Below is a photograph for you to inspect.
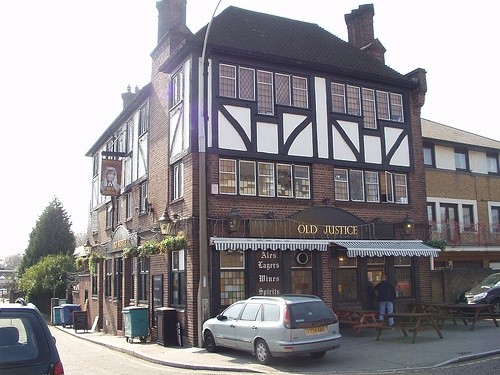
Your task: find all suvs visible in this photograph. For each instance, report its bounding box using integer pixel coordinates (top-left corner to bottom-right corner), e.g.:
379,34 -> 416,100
0,297 -> 64,375
202,292 -> 343,364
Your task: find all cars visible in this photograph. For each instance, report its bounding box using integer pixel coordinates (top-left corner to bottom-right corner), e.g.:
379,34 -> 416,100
464,272 -> 500,321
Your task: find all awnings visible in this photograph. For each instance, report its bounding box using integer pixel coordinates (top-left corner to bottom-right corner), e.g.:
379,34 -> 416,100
213,240 -> 328,252
334,242 -> 441,257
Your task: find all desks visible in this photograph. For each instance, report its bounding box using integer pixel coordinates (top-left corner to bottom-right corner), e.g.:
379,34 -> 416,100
333,307 -> 380,336
375,312 -> 444,345
437,302 -> 500,332
408,302 -> 458,325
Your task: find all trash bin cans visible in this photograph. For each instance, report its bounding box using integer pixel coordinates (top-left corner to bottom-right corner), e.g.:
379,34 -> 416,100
60,304 -> 80,325
121,306 -> 149,336
155,307 -> 177,346
53,307 -> 61,325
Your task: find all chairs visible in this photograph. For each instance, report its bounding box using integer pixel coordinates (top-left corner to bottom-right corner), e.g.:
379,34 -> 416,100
0,326 -> 32,362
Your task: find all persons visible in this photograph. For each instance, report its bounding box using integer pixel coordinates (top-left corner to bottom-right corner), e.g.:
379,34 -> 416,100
372,275 -> 395,326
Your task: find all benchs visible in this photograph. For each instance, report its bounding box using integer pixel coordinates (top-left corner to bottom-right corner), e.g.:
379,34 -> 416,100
338,319 -> 360,330
462,311 -> 499,316
366,319 -> 385,329
376,323 -> 419,333
435,315 -> 478,320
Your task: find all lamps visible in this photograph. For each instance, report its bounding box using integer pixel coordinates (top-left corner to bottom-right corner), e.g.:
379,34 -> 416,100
224,203 -> 244,235
401,212 -> 416,240
157,205 -> 174,235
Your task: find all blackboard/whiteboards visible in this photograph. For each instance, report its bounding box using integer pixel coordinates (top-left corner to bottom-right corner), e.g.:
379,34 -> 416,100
72,310 -> 87,330
